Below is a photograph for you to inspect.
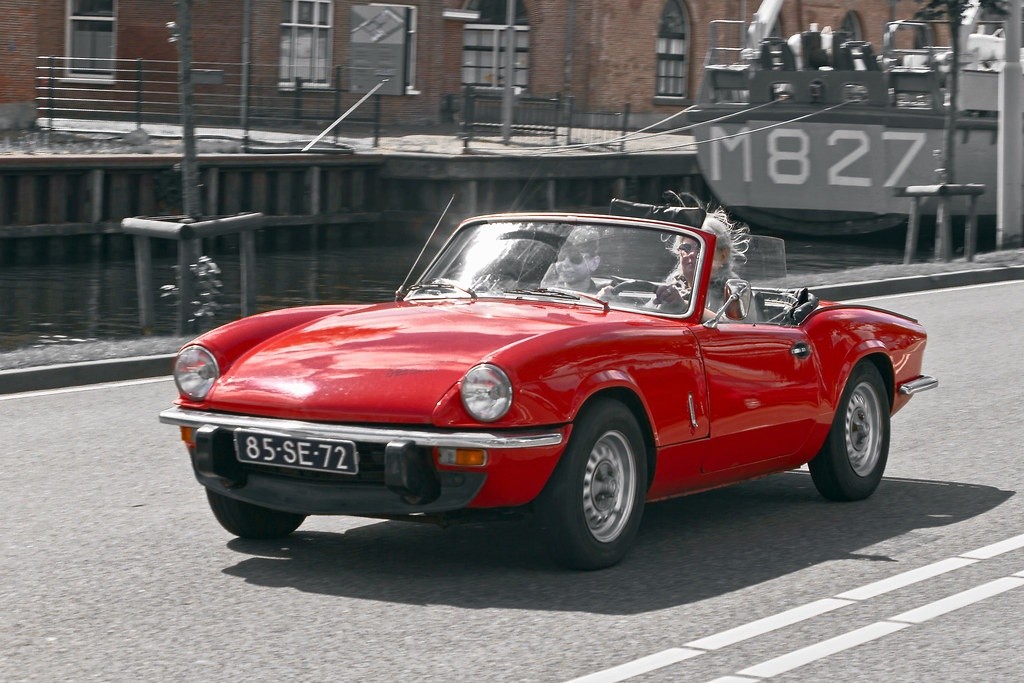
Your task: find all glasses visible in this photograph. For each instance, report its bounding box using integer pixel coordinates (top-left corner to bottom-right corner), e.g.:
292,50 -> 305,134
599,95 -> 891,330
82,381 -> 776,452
553,251 -> 592,264
677,244 -> 701,254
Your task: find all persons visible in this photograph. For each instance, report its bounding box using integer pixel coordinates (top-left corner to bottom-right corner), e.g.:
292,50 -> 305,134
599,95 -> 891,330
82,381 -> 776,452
598,212 -> 756,324
550,225 -> 604,294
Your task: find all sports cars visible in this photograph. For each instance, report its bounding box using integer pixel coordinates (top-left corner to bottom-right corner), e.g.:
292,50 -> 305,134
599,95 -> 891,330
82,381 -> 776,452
156,198 -> 938,572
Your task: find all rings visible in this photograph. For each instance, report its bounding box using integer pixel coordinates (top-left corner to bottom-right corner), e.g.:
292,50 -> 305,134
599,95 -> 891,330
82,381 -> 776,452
666,289 -> 672,293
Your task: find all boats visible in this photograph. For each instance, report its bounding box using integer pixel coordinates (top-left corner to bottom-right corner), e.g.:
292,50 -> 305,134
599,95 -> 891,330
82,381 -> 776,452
684,0 -> 1024,239
